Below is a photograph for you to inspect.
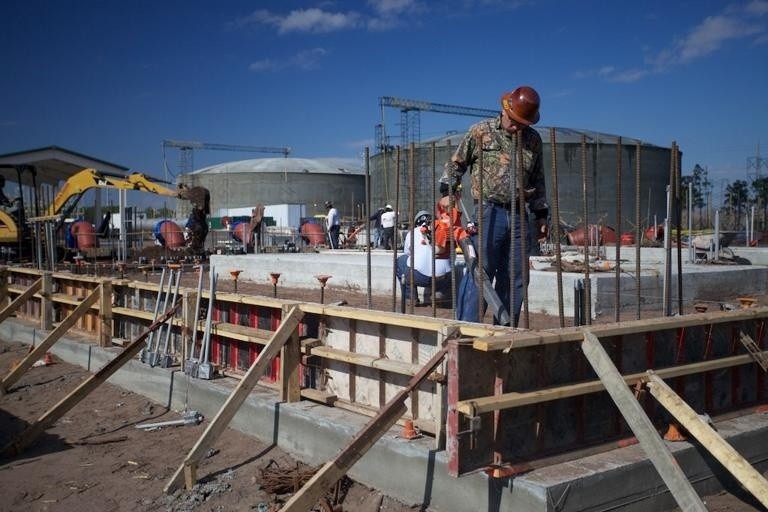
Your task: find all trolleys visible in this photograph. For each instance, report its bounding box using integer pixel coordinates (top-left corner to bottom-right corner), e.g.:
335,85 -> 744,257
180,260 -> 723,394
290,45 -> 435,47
675,231 -> 737,262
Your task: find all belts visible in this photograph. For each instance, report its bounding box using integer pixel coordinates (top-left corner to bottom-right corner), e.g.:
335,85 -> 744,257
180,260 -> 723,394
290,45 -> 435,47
472,198 -> 522,210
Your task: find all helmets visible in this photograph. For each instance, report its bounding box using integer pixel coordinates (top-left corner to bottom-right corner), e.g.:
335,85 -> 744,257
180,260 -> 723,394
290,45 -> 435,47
385,204 -> 393,210
500,86 -> 540,127
324,200 -> 333,209
414,210 -> 432,226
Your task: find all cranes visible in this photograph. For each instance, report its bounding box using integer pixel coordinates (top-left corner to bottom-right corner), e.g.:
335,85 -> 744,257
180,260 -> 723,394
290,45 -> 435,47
155,138 -> 296,222
376,95 -> 505,232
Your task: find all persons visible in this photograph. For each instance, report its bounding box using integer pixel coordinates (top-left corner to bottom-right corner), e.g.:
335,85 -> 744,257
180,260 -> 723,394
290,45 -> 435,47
437,85 -> 551,328
324,200 -> 342,249
368,207 -> 386,249
380,204 -> 401,250
393,210 -> 465,308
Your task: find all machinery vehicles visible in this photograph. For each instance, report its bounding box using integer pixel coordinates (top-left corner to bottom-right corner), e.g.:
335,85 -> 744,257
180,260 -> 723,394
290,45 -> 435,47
0,161 -> 211,262
